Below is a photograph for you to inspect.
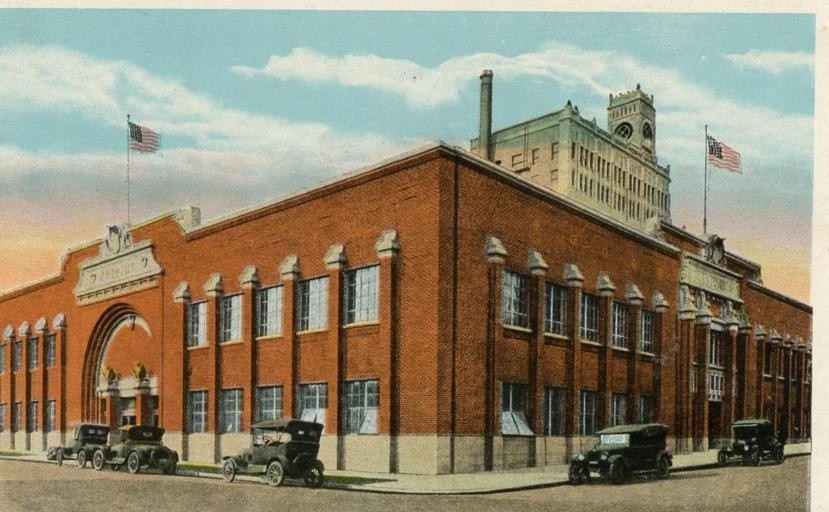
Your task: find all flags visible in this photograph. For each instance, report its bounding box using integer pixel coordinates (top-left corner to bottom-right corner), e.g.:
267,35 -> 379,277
126,117 -> 164,159
703,122 -> 743,176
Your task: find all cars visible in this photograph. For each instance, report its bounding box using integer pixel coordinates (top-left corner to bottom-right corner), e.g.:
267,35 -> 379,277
567,418 -> 672,485
44,422 -> 110,468
90,421 -> 179,476
716,419 -> 787,469
220,417 -> 325,490
